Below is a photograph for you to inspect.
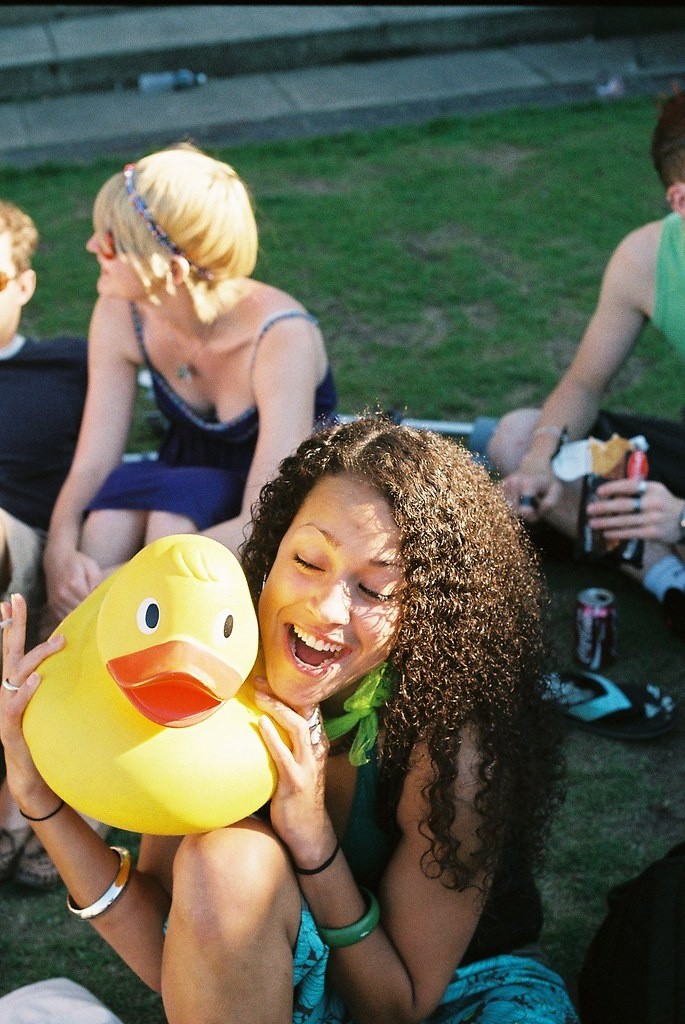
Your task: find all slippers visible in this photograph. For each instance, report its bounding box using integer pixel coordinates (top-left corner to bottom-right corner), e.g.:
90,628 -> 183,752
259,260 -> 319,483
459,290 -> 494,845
558,669 -> 678,740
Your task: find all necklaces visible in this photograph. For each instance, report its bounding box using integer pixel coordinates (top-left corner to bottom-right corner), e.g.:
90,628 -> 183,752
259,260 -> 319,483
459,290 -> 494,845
174,357 -> 197,382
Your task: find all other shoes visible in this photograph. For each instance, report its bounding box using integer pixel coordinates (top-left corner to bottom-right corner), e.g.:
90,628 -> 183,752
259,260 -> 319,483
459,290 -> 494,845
0,817 -> 112,888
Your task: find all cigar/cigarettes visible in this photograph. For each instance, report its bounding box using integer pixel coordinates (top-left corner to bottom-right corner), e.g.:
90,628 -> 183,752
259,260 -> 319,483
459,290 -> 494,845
0,617 -> 12,628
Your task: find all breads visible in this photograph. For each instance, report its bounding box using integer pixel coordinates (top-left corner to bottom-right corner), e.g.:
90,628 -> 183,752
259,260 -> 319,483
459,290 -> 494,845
586,434 -> 637,481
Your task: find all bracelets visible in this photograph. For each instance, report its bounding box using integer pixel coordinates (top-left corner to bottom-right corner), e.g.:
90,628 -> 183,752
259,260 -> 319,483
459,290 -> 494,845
318,887 -> 380,949
530,427 -> 568,443
20,800 -> 65,822
294,840 -> 340,875
66,845 -> 134,922
678,507 -> 685,545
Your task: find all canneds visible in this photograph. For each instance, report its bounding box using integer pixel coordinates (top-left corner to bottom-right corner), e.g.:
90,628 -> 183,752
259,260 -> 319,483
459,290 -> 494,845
574,587 -> 617,671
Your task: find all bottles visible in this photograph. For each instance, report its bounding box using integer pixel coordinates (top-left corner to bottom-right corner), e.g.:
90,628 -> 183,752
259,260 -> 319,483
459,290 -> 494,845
139,68 -> 208,94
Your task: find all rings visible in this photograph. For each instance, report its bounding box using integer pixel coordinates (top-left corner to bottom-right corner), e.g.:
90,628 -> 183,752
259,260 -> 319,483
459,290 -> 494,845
638,481 -> 648,495
307,708 -> 323,746
634,498 -> 641,512
519,495 -> 535,506
3,679 -> 19,692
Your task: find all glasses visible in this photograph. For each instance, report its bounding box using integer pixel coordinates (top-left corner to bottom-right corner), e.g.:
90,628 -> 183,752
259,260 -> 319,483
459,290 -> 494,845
0,271 -> 17,292
95,229 -> 135,260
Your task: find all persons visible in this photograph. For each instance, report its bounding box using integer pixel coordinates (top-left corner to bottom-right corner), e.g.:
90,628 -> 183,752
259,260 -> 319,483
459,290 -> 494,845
0,419 -> 568,1024
42,148 -> 338,623
0,201 -> 90,604
488,89 -> 685,612
